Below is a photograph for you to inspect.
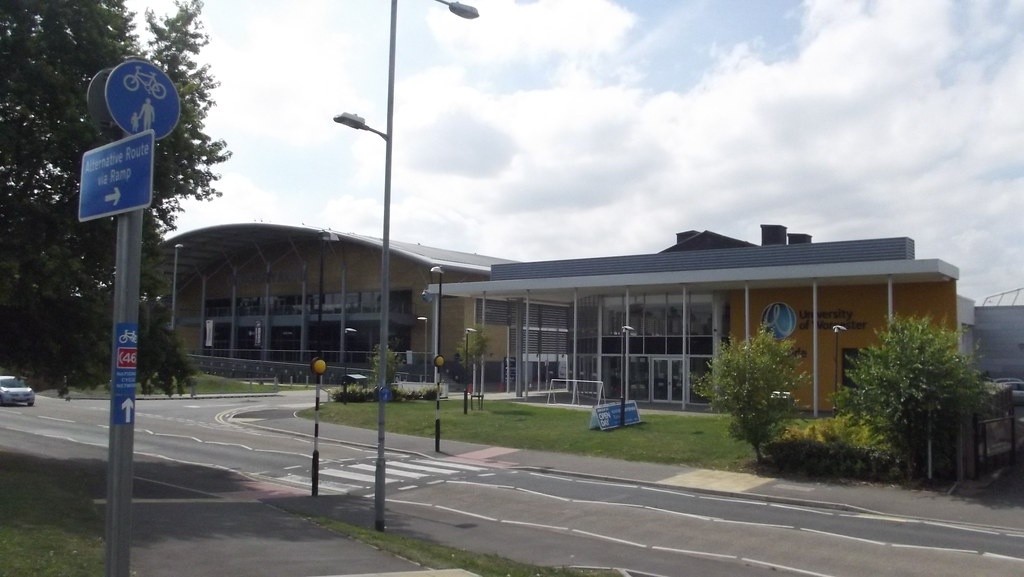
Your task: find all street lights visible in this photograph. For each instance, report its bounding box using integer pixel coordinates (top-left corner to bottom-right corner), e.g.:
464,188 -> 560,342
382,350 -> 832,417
430,267 -> 444,452
170,244 -> 184,330
331,0 -> 479,534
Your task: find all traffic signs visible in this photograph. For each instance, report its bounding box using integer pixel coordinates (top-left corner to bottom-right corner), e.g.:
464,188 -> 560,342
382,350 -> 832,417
78,129 -> 154,223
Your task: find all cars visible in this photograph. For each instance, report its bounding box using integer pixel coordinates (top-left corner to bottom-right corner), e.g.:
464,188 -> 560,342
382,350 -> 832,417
0,376 -> 36,406
